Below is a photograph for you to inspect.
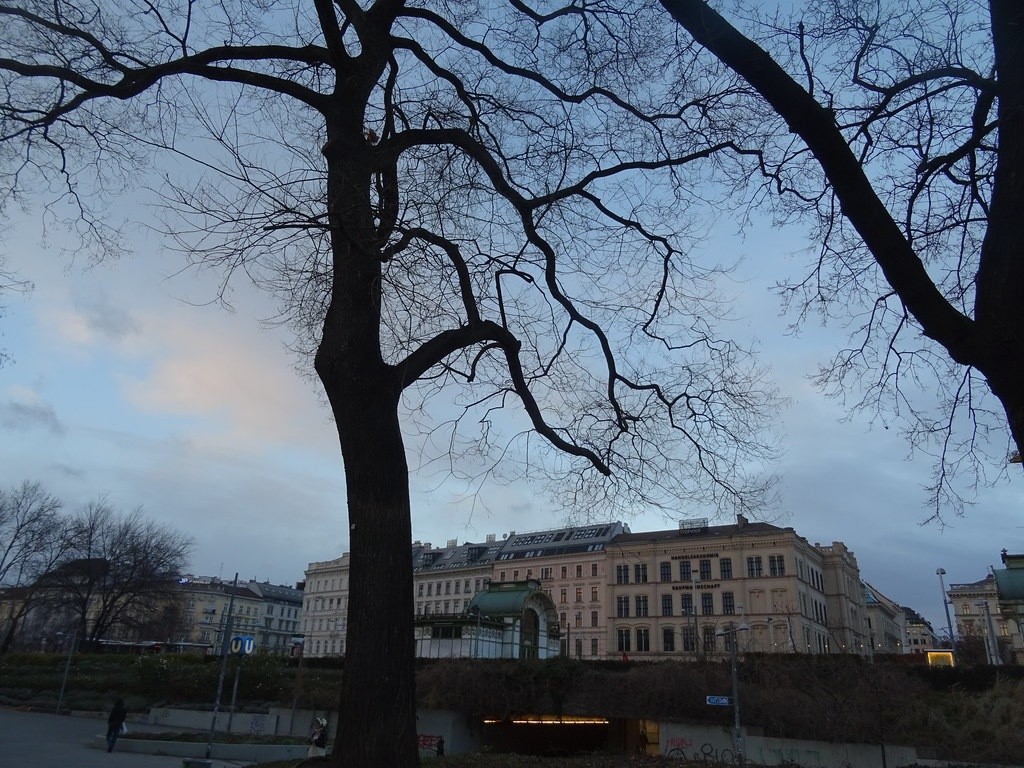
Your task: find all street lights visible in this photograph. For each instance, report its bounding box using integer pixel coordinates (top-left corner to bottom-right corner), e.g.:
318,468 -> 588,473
680,609 -> 694,662
936,567 -> 962,664
974,602 -> 999,665
714,624 -> 750,768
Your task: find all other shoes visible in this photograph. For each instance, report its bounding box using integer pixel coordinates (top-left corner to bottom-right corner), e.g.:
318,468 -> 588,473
107,750 -> 113,752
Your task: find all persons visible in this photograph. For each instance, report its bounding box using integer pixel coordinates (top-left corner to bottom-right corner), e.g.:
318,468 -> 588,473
633,745 -> 640,761
307,717 -> 329,759
106,698 -> 127,753
640,730 -> 650,753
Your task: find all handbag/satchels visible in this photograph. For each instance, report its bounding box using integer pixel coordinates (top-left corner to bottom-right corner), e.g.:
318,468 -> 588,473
119,722 -> 127,735
